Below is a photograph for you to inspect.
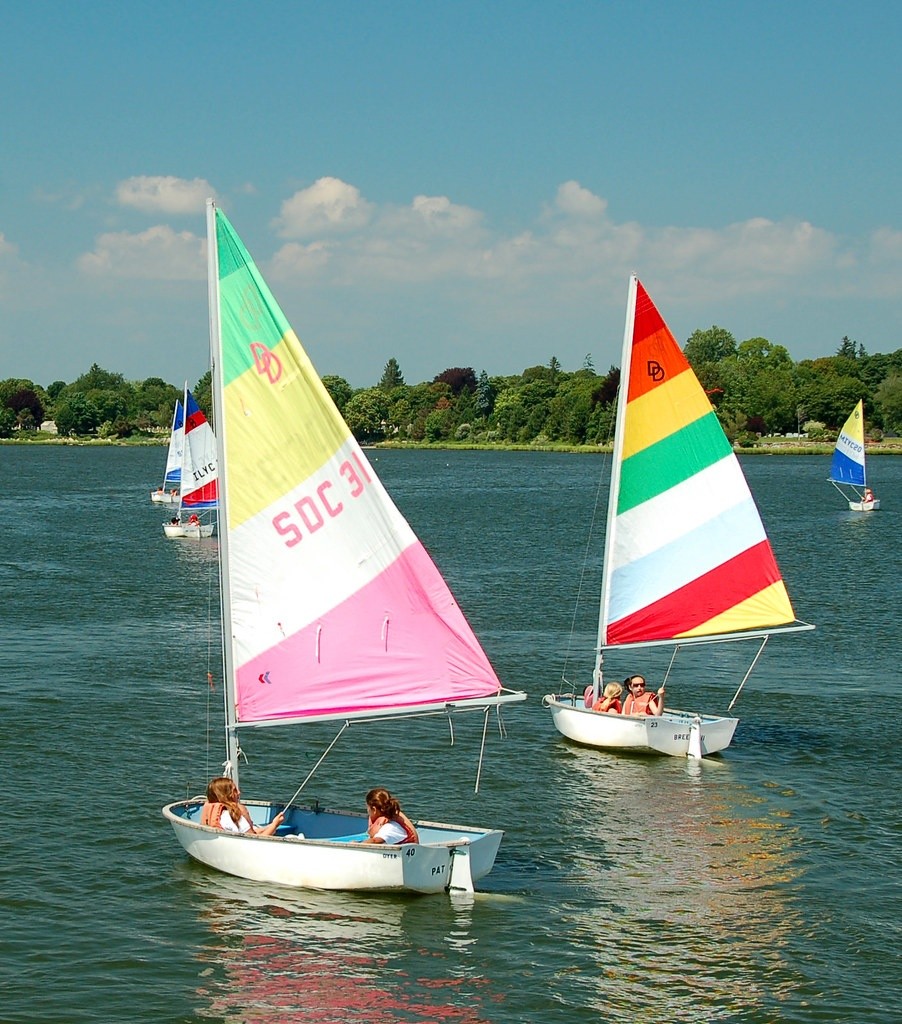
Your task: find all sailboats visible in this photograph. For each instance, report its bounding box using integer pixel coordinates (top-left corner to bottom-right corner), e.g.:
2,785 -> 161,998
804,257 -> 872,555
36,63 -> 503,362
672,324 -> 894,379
162,380 -> 216,537
826,398 -> 881,511
160,199 -> 527,891
539,272 -> 815,765
151,398 -> 184,504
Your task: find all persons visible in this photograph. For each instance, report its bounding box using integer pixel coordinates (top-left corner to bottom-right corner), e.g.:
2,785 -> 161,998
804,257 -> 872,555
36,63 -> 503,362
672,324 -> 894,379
350,787 -> 420,845
170,489 -> 177,496
592,682 -> 622,714
864,489 -> 874,503
157,487 -> 163,495
188,514 -> 200,526
171,517 -> 181,525
621,675 -> 665,716
201,777 -> 284,836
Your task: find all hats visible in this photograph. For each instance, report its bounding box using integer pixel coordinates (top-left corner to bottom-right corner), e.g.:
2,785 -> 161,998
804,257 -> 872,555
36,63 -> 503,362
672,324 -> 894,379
866,488 -> 871,493
171,517 -> 180,523
189,514 -> 198,521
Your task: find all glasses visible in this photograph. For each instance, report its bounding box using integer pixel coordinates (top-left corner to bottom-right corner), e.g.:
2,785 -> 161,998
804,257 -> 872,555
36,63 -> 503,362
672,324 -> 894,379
631,682 -> 645,687
